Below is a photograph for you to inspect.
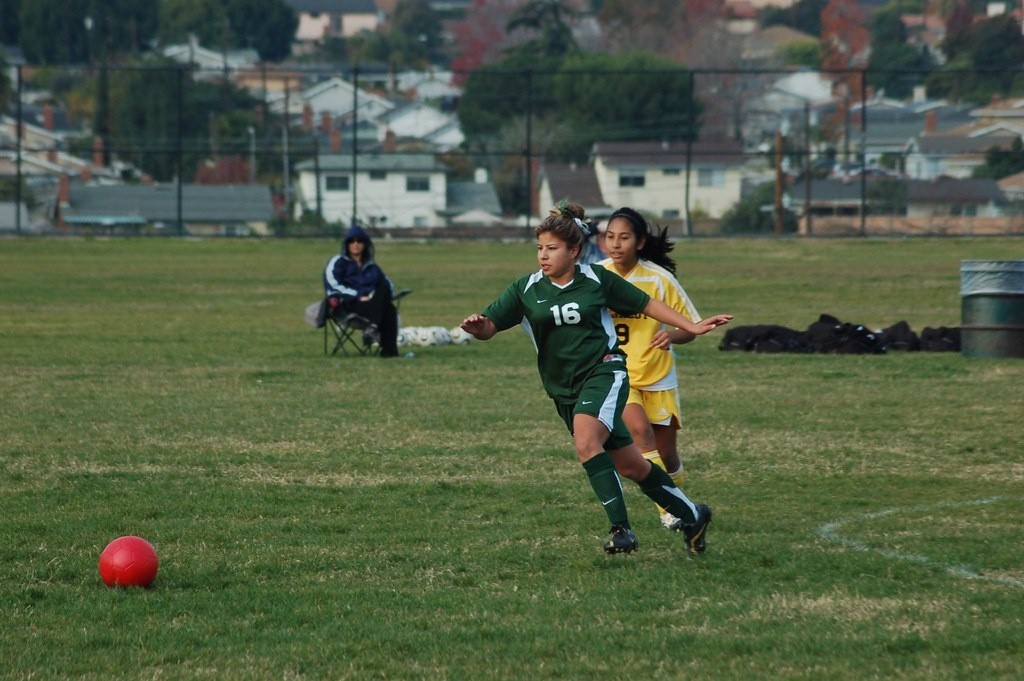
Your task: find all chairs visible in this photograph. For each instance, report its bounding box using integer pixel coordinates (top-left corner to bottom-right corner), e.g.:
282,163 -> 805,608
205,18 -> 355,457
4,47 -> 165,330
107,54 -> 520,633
323,269 -> 400,356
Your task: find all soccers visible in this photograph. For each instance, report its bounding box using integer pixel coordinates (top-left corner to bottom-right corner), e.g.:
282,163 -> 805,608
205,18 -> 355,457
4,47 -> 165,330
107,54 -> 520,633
450,326 -> 474,347
397,326 -> 449,346
100,536 -> 159,586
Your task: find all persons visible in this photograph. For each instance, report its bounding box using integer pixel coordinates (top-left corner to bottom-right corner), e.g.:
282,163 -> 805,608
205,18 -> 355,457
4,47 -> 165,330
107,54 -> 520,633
322,224 -> 401,358
591,206 -> 701,531
460,203 -> 734,559
578,218 -> 611,267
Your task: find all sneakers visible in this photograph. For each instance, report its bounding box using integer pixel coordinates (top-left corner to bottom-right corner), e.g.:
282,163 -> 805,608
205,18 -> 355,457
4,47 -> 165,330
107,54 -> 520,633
603,524 -> 638,554
680,503 -> 711,557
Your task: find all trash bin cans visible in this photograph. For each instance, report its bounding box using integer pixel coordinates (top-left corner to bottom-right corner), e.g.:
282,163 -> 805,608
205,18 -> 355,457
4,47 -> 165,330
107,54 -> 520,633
960,259 -> 1024,360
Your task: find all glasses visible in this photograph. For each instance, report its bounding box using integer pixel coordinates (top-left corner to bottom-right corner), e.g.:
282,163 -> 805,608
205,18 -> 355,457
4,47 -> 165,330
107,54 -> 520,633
348,239 -> 362,244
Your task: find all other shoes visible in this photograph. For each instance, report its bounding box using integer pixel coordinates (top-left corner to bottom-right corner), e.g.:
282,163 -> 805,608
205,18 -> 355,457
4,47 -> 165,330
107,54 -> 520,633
362,330 -> 373,346
661,513 -> 682,528
